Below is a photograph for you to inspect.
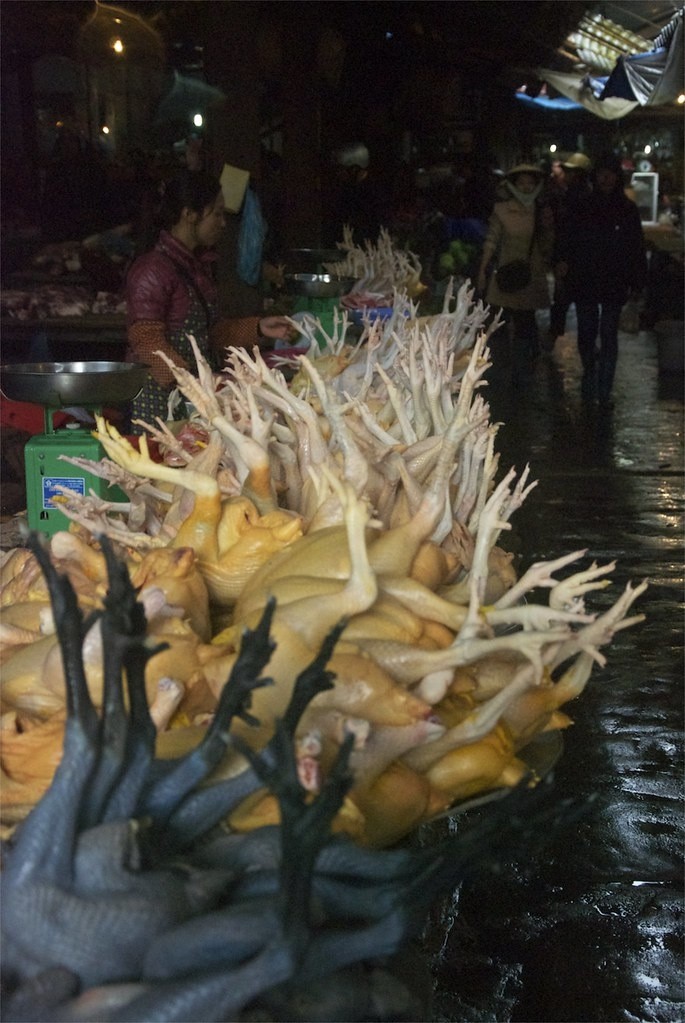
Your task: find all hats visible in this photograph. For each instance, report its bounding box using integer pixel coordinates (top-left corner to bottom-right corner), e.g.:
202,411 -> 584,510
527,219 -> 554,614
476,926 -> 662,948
507,164 -> 542,174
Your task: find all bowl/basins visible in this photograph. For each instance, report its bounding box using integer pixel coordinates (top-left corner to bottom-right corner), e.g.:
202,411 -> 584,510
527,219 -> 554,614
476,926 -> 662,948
354,307 -> 407,326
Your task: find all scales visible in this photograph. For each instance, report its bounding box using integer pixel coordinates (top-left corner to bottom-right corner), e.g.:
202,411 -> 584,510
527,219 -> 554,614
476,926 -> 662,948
285,246 -> 350,314
0,358 -> 151,541
283,272 -> 364,355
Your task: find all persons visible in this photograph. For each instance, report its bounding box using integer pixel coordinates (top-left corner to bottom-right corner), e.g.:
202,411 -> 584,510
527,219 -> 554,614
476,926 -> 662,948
35,132 -> 615,271
559,154 -> 650,412
475,160 -> 553,384
122,172 -> 292,454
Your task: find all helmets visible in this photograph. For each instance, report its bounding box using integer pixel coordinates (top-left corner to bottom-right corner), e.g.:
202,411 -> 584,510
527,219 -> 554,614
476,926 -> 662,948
332,139 -> 369,172
563,152 -> 590,170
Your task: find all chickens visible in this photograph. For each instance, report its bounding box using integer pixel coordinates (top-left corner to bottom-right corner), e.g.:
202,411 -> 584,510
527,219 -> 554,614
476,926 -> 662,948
0,222 -> 650,1023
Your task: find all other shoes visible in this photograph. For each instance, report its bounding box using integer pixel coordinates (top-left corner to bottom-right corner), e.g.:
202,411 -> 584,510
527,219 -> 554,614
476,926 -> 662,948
579,377 -> 616,412
542,330 -> 559,352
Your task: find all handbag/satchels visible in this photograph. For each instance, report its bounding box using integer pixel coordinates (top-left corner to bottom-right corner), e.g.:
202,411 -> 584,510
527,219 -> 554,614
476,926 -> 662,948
496,260 -> 532,291
